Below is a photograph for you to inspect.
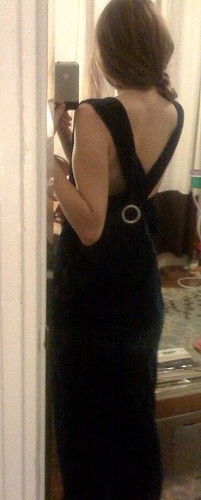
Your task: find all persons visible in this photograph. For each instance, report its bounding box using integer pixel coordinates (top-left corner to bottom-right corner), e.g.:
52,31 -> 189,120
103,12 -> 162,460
47,1 -> 185,499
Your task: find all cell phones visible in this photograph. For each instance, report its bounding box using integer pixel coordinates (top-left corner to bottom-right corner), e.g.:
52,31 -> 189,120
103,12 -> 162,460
54,61 -> 79,110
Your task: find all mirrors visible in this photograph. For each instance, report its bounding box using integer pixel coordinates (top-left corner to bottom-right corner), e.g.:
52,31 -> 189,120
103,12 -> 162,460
43,1 -> 201,500
